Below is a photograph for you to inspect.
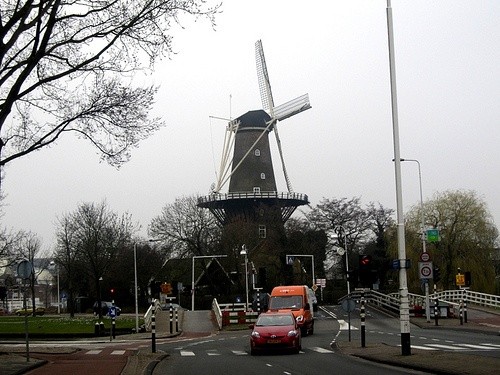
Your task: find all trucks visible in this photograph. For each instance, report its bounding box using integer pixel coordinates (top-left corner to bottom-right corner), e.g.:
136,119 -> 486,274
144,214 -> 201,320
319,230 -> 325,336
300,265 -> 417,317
266,286 -> 316,337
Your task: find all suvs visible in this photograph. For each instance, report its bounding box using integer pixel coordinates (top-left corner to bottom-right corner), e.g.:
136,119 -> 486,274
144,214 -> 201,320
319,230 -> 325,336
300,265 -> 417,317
93,301 -> 121,316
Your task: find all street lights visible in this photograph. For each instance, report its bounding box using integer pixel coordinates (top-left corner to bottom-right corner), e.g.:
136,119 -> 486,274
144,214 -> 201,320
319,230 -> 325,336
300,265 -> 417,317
239,244 -> 250,311
392,158 -> 433,323
133,239 -> 160,332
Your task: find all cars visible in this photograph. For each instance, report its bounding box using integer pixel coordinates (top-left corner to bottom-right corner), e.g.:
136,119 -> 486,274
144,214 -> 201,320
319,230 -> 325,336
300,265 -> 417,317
248,312 -> 302,354
309,289 -> 317,305
15,304 -> 46,316
0,308 -> 12,315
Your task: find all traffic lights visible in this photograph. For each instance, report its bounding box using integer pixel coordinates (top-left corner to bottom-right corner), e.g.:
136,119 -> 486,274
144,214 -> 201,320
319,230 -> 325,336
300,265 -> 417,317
433,266 -> 441,284
349,251 -> 379,279
